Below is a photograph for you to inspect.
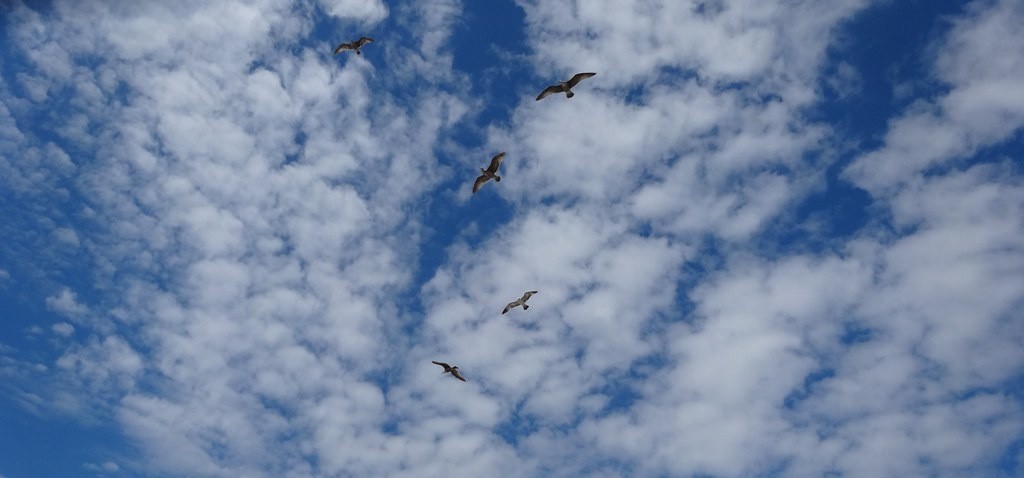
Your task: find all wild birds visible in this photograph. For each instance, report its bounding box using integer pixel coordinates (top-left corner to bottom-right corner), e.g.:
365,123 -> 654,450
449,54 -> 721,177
536,71 -> 596,103
334,38 -> 375,57
430,291 -> 544,383
473,151 -> 508,193
